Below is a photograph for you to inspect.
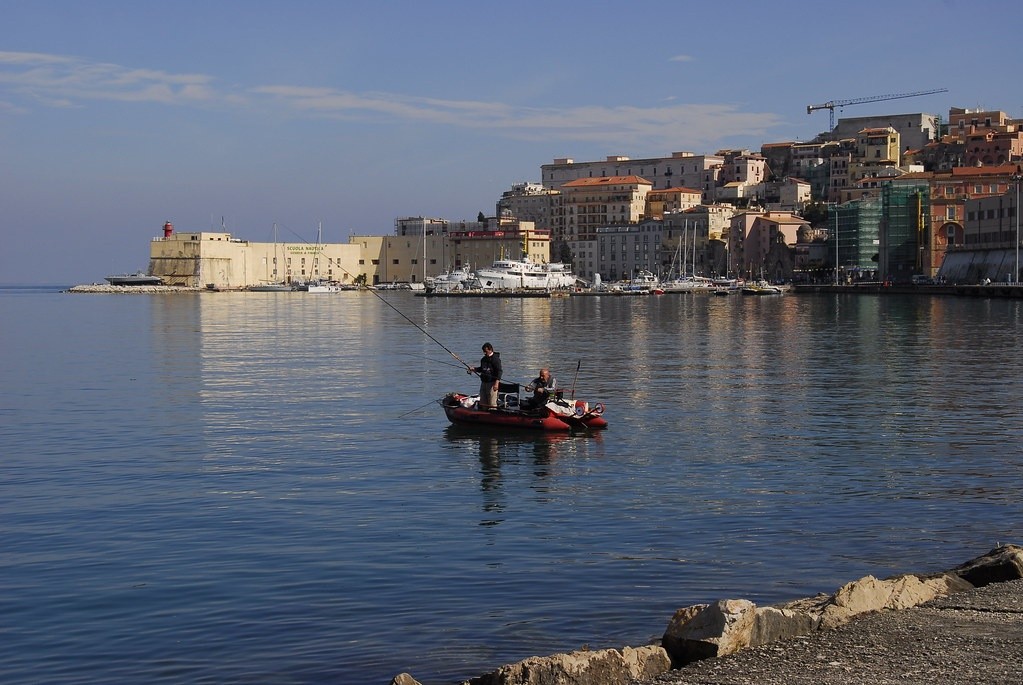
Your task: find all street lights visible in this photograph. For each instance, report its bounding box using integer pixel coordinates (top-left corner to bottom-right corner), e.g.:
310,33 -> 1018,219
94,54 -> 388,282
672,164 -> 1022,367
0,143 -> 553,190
831,202 -> 840,285
1009,174 -> 1023,284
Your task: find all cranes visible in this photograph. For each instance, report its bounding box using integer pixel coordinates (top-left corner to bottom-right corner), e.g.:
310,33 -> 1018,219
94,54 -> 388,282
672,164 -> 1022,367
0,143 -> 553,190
807,87 -> 950,134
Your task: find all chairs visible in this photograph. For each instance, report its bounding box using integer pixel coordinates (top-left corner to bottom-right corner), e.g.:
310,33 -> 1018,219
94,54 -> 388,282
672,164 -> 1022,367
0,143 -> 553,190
498,382 -> 520,408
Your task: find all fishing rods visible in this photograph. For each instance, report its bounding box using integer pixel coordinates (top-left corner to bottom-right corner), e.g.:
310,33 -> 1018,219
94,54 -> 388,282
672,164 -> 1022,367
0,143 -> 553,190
383,349 -> 535,392
277,223 -> 481,380
397,393 -> 454,419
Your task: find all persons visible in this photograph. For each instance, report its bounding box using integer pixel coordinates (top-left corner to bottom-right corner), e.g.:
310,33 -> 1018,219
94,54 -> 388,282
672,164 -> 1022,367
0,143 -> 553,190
468,343 -> 502,411
520,368 -> 557,418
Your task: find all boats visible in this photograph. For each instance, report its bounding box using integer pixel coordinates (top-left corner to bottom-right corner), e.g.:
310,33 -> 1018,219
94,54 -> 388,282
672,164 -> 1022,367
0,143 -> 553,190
248,217 -> 794,300
104,268 -> 164,285
435,358 -> 609,434
